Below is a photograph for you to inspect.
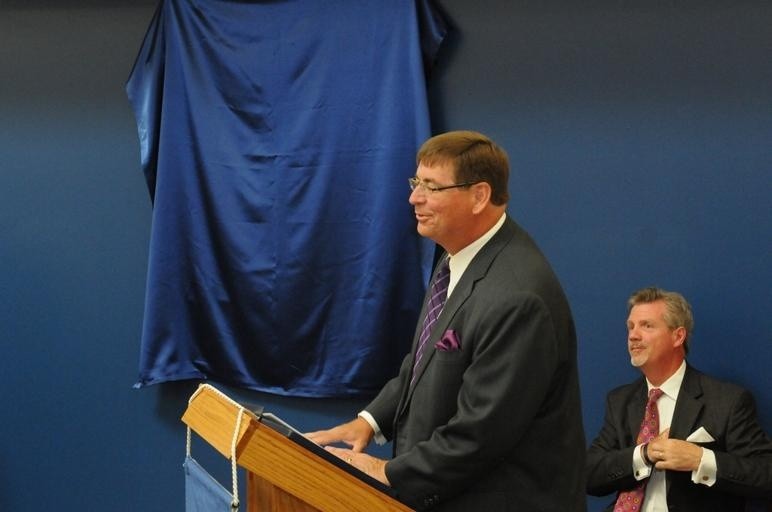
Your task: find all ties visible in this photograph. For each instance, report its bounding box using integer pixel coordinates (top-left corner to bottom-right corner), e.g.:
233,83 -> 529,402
613,388 -> 663,512
409,257 -> 451,391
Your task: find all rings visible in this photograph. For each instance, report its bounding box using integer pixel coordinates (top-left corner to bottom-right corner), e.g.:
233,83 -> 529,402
658,451 -> 664,460
347,457 -> 352,465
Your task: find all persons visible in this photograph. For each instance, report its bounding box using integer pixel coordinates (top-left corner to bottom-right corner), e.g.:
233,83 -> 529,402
303,131 -> 588,511
584,288 -> 771,511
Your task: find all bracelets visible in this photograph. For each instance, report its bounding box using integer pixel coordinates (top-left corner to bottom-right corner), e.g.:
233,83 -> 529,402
643,441 -> 655,466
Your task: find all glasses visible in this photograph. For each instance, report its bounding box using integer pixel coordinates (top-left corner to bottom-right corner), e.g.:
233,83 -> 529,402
408,177 -> 474,197
